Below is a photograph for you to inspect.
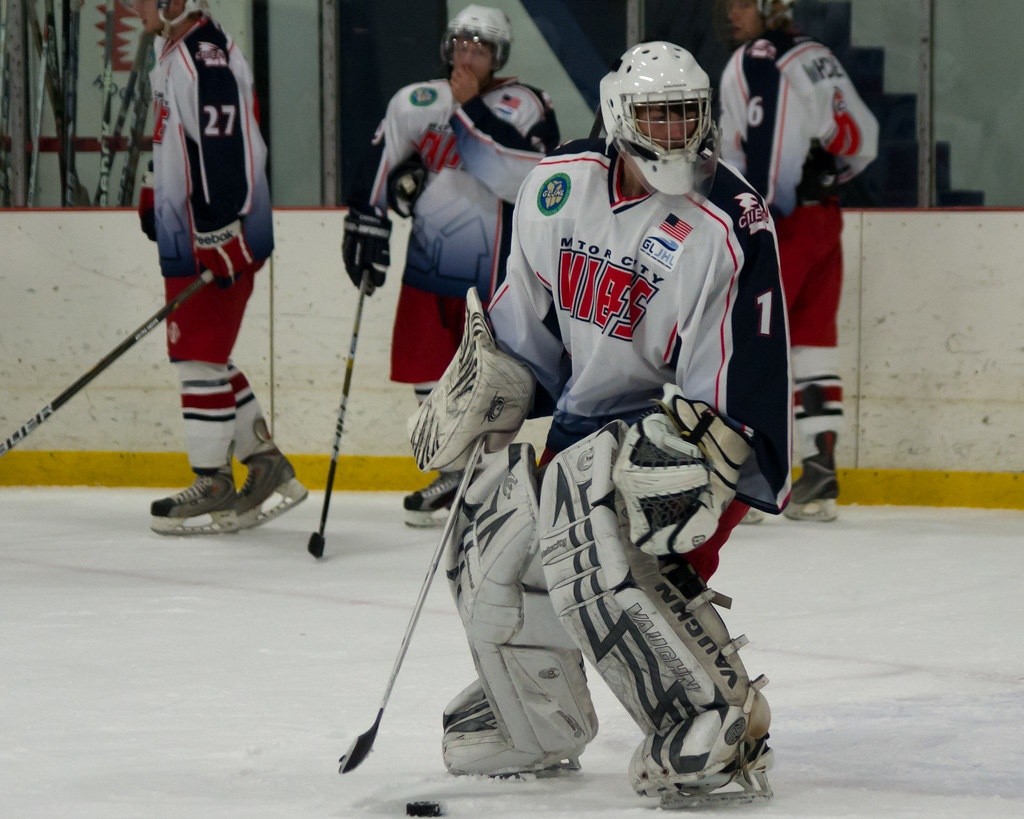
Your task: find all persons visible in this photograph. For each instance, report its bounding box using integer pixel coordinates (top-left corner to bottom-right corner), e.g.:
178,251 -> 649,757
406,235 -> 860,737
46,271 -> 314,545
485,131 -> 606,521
131,0 -> 311,535
719,0 -> 881,522
342,3 -> 560,530
408,42 -> 792,812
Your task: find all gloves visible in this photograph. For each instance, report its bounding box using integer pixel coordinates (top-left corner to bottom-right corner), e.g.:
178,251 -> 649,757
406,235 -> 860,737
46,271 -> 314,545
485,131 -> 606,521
342,208 -> 392,296
386,151 -> 426,218
188,219 -> 256,288
794,137 -> 850,207
138,159 -> 157,241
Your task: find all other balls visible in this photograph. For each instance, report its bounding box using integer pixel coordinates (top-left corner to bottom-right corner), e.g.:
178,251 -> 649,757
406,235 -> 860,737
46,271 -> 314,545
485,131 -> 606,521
406,801 -> 440,817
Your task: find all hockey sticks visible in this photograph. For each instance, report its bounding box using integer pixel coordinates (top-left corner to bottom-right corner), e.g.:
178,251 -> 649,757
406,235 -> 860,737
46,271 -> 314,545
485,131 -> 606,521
336,430 -> 489,775
0,268 -> 216,457
307,266 -> 371,560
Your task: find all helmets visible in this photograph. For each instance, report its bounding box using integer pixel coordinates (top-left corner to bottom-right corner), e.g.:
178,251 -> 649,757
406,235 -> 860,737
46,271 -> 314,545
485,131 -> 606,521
600,40 -> 713,196
755,0 -> 796,21
447,4 -> 513,72
153,0 -> 203,26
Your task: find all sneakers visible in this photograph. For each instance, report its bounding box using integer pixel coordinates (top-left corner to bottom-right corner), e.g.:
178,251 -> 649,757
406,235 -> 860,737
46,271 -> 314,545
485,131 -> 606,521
782,430 -> 840,521
739,507 -> 764,524
644,732 -> 774,809
235,416 -> 308,529
488,746 -> 582,780
149,440 -> 242,536
403,472 -> 473,526
